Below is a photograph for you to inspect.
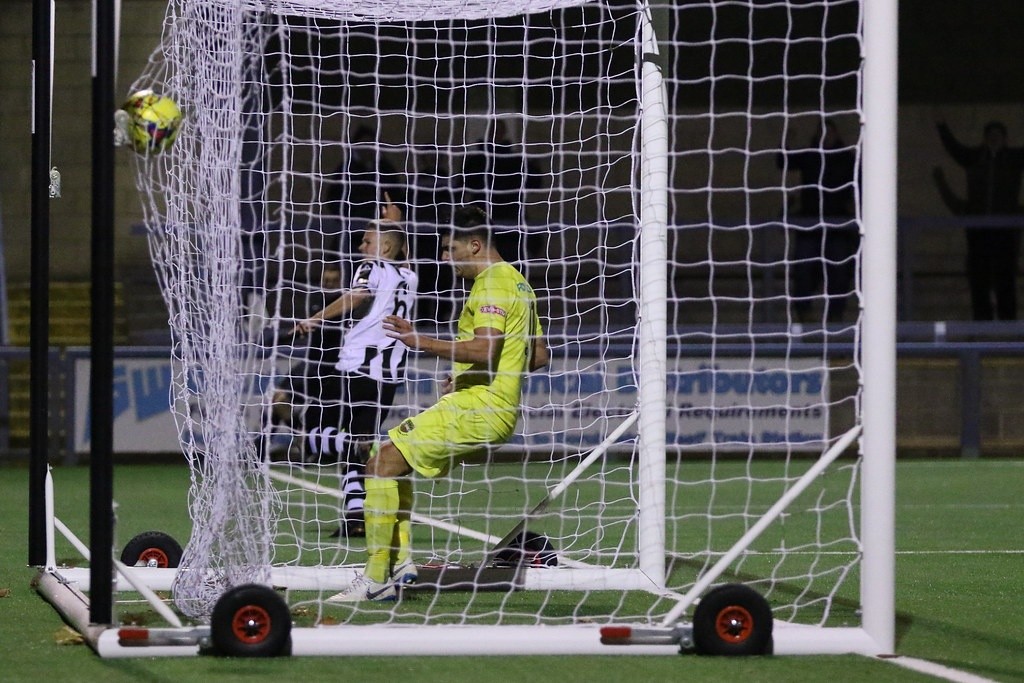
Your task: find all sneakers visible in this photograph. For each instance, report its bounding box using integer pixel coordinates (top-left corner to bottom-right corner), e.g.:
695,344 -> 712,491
330,512 -> 366,536
326,569 -> 397,602
352,557 -> 417,591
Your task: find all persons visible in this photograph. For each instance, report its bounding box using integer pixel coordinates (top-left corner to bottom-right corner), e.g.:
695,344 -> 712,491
328,206 -> 546,602
323,120 -> 544,260
308,267 -> 353,360
777,118 -> 855,319
929,103 -> 1023,322
242,191 -> 420,538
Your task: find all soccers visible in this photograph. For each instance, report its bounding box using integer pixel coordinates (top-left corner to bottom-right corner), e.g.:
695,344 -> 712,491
120,89 -> 182,157
